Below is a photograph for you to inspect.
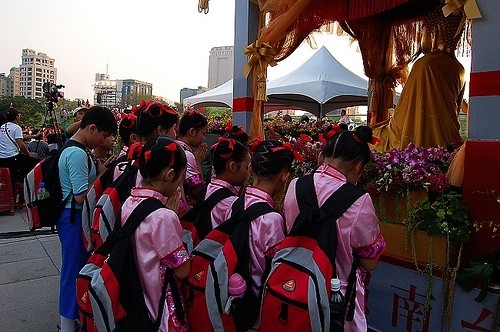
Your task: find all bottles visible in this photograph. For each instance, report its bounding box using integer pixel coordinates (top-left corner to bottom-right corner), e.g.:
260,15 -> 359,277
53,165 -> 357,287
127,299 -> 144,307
229,273 -> 247,299
329,278 -> 346,332
37,182 -> 46,200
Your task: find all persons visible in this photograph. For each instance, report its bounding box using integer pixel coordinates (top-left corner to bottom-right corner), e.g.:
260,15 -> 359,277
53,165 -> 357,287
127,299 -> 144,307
27,129 -> 58,160
0,107 -> 38,169
77,99 -> 89,107
284,124 -> 387,332
368,0 -> 467,153
113,99 -> 208,218
121,137 -> 190,332
203,136 -> 251,229
339,110 -> 350,125
55,106 -> 118,332
224,136 -> 306,297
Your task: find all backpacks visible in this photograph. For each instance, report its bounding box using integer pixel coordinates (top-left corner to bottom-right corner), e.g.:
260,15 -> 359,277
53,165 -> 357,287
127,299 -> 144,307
24,140 -> 92,232
180,183 -> 284,332
81,153 -> 141,254
76,198 -> 184,332
258,172 -> 367,332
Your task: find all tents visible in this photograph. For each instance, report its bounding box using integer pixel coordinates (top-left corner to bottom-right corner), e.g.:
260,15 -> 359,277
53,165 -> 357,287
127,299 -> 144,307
183,46 -> 400,119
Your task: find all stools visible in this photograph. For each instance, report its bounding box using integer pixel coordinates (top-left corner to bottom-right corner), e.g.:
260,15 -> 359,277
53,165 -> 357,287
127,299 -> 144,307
0,168 -> 15,216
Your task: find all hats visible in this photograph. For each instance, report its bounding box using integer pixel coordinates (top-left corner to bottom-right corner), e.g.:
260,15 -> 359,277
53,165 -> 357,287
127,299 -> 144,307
72,107 -> 89,116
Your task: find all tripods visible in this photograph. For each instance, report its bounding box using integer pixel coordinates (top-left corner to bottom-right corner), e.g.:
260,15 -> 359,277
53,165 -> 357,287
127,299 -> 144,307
35,103 -> 63,153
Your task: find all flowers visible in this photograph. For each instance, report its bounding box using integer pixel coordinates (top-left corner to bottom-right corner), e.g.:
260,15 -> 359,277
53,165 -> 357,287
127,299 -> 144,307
247,109 -> 472,332
